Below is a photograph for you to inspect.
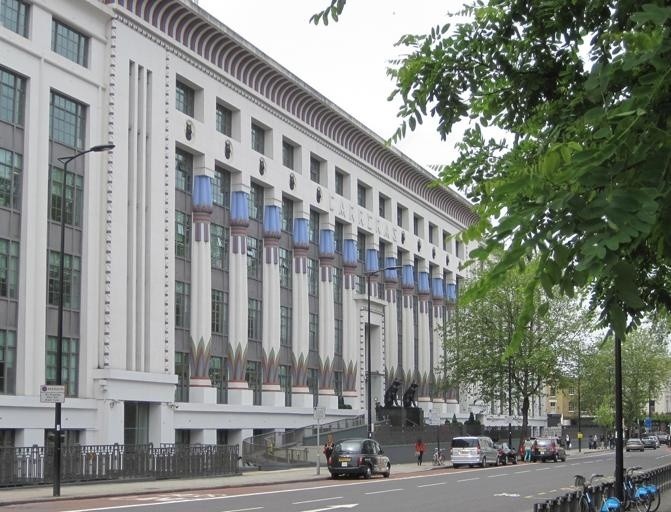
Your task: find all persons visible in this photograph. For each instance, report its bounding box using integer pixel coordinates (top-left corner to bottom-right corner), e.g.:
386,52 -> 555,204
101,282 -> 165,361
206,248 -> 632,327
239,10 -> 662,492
323,440 -> 335,462
555,434 -> 616,449
415,440 -> 425,466
524,439 -> 532,462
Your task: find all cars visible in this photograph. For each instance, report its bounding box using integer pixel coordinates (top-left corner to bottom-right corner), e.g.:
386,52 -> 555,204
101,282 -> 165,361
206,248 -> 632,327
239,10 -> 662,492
626,430 -> 671,451
519,436 -> 543,461
328,437 -> 391,479
495,442 -> 518,465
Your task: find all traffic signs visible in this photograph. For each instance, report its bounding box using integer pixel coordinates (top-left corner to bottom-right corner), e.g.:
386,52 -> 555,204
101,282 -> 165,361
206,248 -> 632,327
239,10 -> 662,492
314,407 -> 325,419
39,385 -> 65,403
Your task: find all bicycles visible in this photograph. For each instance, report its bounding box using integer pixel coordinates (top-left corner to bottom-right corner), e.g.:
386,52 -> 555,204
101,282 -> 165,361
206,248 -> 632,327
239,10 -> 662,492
573,465 -> 661,511
432,447 -> 449,466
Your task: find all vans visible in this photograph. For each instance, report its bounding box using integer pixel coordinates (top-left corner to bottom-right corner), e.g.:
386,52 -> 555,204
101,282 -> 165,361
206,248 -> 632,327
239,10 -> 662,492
451,436 -> 501,468
531,436 -> 566,462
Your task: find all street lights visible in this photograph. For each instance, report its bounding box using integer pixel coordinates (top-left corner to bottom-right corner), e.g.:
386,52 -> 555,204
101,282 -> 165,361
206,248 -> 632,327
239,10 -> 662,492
366,266 -> 406,439
53,142 -> 116,497
509,324 -> 531,450
578,354 -> 594,452
